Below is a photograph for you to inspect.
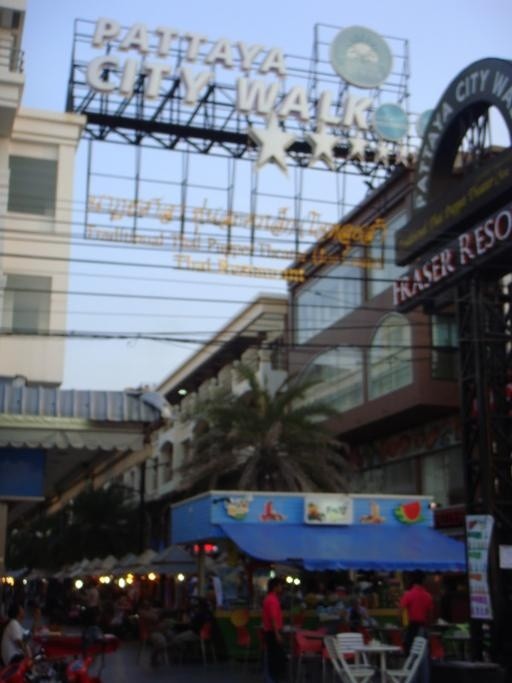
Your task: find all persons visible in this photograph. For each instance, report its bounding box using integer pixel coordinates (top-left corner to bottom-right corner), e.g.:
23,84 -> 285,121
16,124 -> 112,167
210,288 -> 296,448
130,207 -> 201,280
79,576 -> 101,641
143,596 -> 214,665
2,603 -> 41,668
344,597 -> 367,626
400,576 -> 437,658
261,576 -> 286,683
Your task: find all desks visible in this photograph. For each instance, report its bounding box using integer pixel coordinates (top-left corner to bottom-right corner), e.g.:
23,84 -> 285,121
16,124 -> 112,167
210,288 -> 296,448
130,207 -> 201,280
32,632 -> 120,683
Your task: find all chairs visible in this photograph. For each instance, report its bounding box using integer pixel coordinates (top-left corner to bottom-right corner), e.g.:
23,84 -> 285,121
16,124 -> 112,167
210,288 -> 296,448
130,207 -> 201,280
232,622 -> 430,682
136,618 -> 216,670
